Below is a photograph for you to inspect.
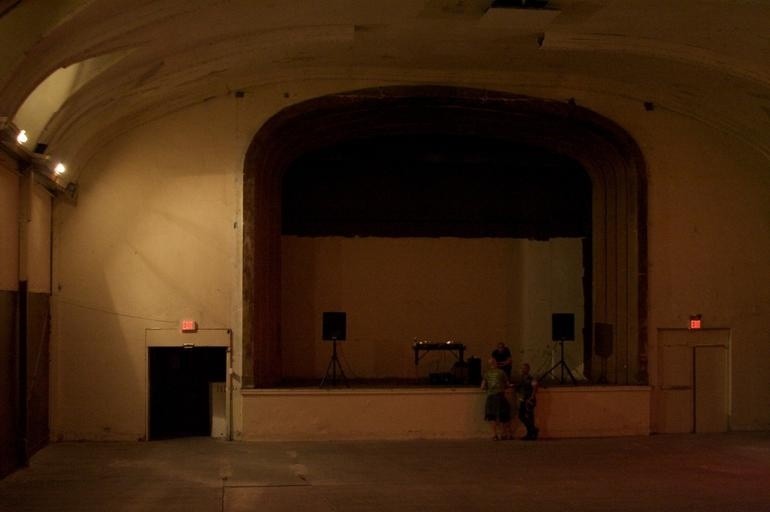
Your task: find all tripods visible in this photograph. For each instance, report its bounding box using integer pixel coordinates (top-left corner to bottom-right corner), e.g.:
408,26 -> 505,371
538,341 -> 578,385
317,344 -> 349,388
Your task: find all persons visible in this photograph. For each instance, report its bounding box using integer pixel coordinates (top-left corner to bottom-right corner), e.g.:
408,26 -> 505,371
492,343 -> 513,387
514,364 -> 539,441
480,358 -> 514,440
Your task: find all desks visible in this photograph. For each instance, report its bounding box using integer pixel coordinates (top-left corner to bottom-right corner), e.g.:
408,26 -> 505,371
412,341 -> 466,383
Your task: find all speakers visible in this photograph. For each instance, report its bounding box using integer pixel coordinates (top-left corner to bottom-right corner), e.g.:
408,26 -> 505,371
551,313 -> 575,341
322,311 -> 347,341
